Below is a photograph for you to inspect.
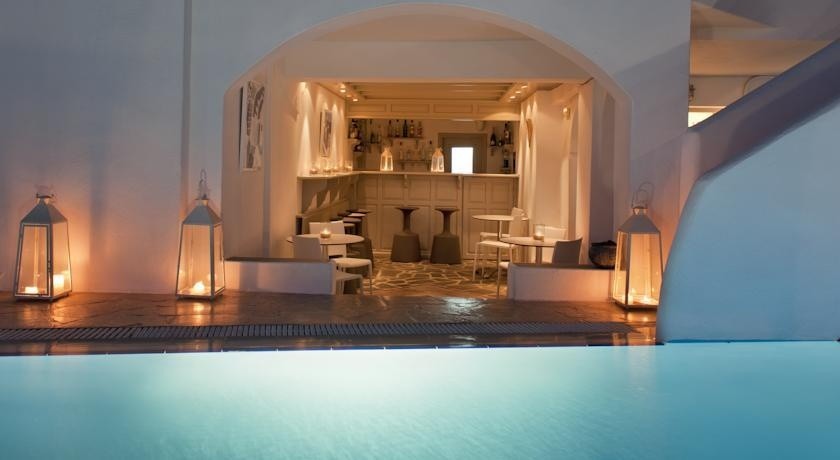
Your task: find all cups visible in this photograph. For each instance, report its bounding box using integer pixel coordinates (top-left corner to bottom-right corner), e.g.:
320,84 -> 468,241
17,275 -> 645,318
533,224 -> 546,239
310,159 -> 354,176
320,219 -> 332,238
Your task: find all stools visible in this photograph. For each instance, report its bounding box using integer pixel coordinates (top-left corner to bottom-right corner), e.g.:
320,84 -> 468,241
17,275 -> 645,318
329,207 -> 374,293
392,206 -> 422,262
431,207 -> 462,264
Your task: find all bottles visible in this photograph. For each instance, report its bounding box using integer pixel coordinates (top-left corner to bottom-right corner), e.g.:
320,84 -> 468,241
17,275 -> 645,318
377,125 -> 382,143
370,132 -> 375,143
399,139 -> 434,161
490,127 -> 497,146
388,119 -> 423,138
499,136 -> 503,146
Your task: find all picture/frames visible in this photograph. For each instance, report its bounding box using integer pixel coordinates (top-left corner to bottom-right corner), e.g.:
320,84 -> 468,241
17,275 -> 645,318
320,110 -> 333,157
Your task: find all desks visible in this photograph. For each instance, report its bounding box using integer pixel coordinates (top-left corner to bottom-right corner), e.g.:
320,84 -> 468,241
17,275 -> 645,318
472,215 -> 529,278
501,237 -> 567,263
286,233 -> 364,263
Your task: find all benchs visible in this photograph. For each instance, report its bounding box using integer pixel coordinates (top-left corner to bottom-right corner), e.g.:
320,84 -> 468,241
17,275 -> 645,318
506,264 -> 626,301
220,256 -> 333,294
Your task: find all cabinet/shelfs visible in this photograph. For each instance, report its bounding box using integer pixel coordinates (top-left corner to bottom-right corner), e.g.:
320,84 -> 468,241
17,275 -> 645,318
500,143 -> 513,172
348,136 -> 432,164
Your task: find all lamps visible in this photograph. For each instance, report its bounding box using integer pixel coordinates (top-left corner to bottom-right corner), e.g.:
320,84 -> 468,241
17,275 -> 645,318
611,182 -> 663,310
12,186 -> 73,303
175,169 -> 225,300
380,145 -> 393,171
430,147 -> 445,172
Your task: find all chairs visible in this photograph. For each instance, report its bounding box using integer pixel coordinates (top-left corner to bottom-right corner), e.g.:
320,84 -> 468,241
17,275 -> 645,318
472,207 -> 583,285
292,220 -> 373,295
